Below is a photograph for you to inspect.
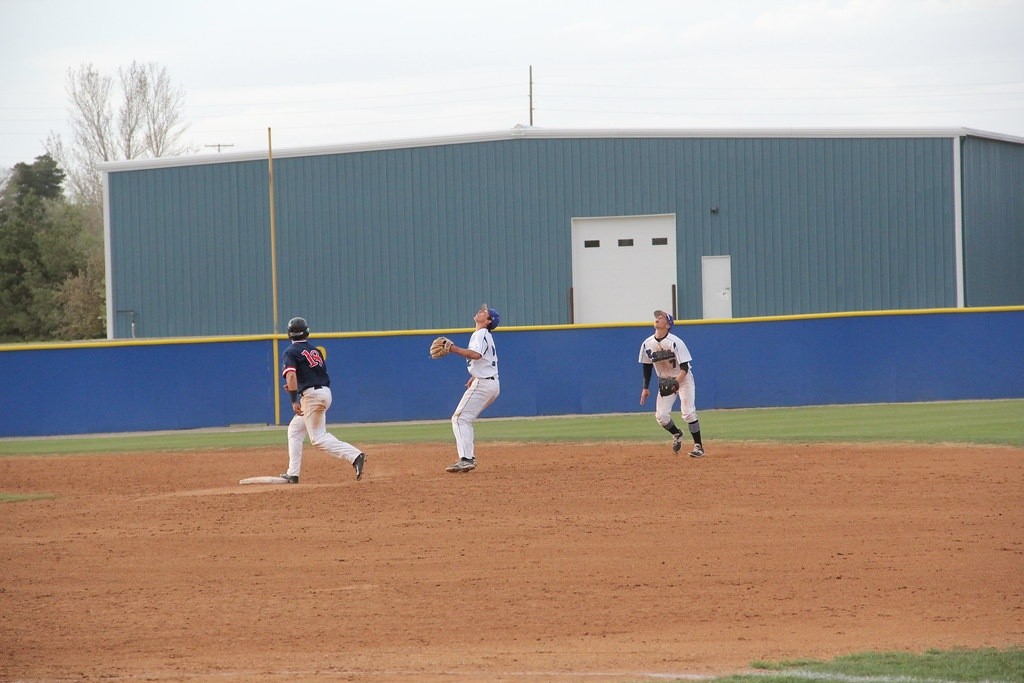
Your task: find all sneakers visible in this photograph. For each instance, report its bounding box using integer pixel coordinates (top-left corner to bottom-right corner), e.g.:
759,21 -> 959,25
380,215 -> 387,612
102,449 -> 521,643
445,458 -> 475,473
688,444 -> 705,458
460,458 -> 478,473
279,472 -> 298,484
672,429 -> 684,454
352,452 -> 368,480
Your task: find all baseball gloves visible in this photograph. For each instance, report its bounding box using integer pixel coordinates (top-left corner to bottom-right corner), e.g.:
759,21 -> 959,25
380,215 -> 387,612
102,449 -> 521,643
429,335 -> 454,359
658,378 -> 679,399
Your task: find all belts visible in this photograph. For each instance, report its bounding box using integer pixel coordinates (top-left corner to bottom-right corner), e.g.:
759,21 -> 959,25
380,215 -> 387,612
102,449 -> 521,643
483,377 -> 494,380
300,385 -> 322,397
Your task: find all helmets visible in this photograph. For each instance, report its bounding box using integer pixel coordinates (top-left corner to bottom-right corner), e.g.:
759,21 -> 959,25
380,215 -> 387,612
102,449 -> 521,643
287,317 -> 310,339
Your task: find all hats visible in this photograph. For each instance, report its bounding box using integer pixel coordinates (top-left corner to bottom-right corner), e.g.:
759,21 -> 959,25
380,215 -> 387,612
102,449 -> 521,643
654,310 -> 674,327
481,303 -> 501,329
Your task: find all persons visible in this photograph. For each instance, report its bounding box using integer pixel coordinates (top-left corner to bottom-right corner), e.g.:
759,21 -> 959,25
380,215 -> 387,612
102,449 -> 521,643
429,303 -> 500,473
279,317 -> 366,484
638,310 -> 705,457
